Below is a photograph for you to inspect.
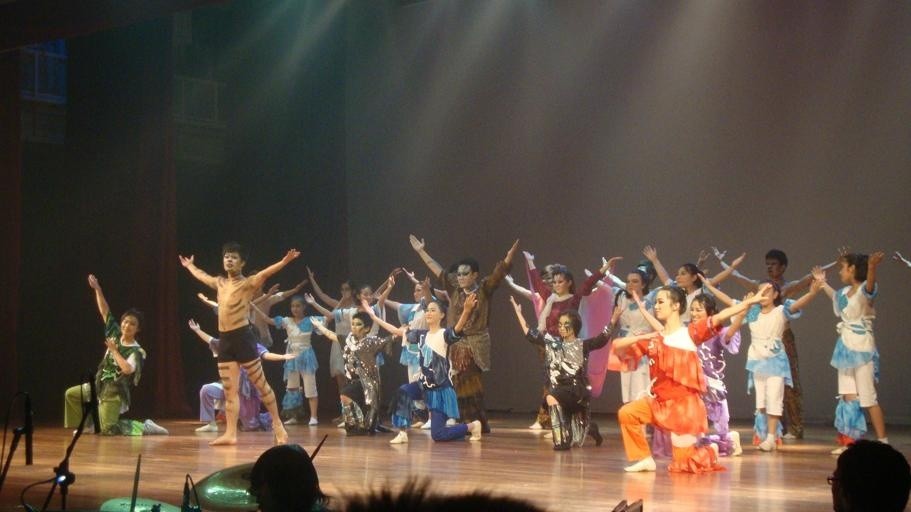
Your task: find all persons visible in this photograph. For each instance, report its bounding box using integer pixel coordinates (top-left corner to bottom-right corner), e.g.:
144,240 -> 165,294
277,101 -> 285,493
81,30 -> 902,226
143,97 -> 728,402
505,245 -> 911,473
241,443 -> 335,512
825,438 -> 910,511
316,473 -> 549,512
64,274 -> 169,436
180,236 -> 519,445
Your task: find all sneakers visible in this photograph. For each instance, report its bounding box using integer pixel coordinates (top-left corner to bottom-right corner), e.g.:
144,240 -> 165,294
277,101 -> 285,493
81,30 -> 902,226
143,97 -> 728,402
530,422 -> 602,451
284,418 -> 297,425
759,442 -> 777,451
728,430 -> 743,455
624,460 -> 657,471
308,417 -> 318,425
195,425 -> 218,432
389,420 -> 482,444
144,419 -> 169,434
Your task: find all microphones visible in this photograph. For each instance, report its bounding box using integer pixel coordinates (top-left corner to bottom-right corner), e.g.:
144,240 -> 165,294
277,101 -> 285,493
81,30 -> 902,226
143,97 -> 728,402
25,397 -> 33,464
180,481 -> 191,512
90,374 -> 101,434
104,337 -> 117,360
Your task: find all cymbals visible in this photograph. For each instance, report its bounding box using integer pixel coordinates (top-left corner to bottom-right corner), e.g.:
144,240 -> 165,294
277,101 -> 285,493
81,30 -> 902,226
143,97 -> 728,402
190,462 -> 260,510
100,498 -> 181,511
